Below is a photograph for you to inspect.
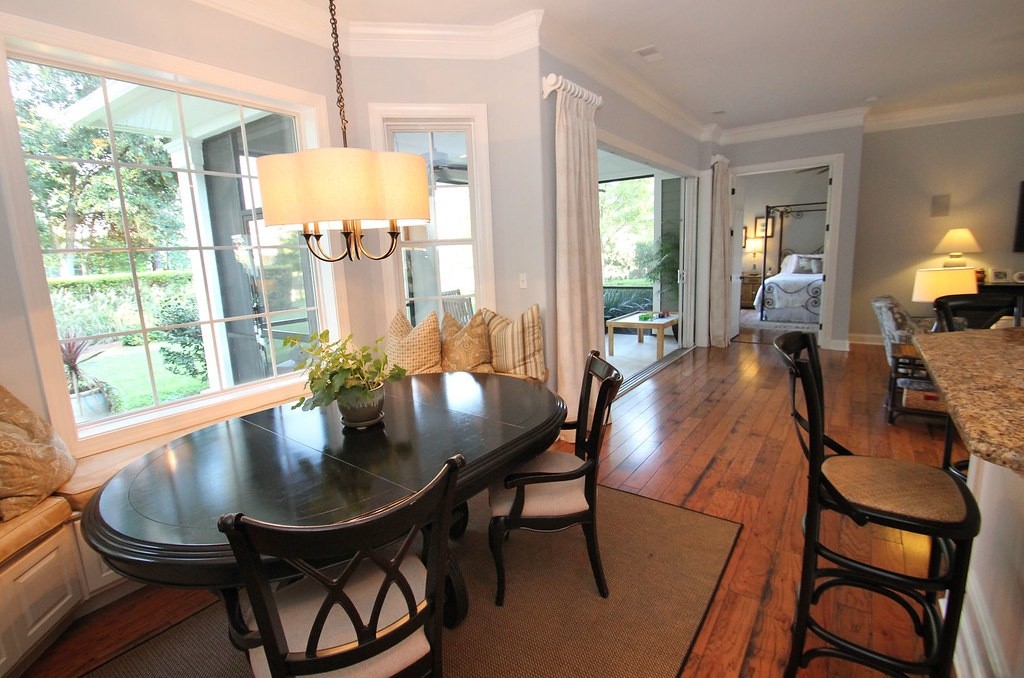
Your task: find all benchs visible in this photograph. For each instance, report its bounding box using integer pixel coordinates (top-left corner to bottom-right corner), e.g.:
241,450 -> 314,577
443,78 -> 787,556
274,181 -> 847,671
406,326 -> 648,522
0,392 -> 319,678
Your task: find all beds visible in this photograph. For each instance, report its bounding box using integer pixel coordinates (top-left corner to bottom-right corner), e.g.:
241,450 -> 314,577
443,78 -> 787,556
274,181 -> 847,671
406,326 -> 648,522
753,201 -> 828,325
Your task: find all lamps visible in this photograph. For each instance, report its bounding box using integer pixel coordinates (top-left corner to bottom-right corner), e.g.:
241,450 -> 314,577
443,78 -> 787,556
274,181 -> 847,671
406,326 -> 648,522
933,226 -> 983,268
911,267 -> 979,330
745,238 -> 764,276
255,0 -> 430,262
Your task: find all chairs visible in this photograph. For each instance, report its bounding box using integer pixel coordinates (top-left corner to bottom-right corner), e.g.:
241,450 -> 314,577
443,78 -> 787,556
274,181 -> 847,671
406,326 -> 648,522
216,454 -> 466,678
772,330 -> 982,678
933,291 -> 1017,332
486,347 -> 623,607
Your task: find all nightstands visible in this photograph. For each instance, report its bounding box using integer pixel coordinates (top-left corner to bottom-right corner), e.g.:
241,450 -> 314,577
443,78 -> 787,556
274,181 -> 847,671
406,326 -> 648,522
740,273 -> 775,310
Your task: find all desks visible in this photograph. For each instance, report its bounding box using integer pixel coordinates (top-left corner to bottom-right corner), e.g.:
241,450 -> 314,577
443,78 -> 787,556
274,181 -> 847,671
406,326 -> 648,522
80,370 -> 569,630
979,281 -> 1024,327
606,310 -> 679,361
885,342 -> 951,426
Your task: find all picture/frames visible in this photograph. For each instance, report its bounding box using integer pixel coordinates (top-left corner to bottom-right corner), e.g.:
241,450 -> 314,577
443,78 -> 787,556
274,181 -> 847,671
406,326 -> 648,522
990,266 -> 1014,283
754,215 -> 774,239
742,226 -> 747,248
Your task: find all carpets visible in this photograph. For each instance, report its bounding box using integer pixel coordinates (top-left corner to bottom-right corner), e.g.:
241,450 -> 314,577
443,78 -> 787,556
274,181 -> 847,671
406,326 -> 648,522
77,481 -> 741,678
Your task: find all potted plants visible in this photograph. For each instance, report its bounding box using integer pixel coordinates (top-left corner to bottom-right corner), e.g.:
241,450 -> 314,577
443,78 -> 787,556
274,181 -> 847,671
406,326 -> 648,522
645,216 -> 684,344
52,326 -> 111,424
283,329 -> 407,427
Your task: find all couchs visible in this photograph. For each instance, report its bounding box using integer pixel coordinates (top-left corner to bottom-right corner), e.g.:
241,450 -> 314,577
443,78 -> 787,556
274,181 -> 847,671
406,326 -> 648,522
870,295 -> 968,414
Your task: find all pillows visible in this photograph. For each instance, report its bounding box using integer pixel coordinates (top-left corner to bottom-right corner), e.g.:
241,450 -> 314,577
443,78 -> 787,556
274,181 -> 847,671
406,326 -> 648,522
439,307 -> 496,373
384,309 -> 442,374
792,255 -> 823,274
482,303 -> 547,385
